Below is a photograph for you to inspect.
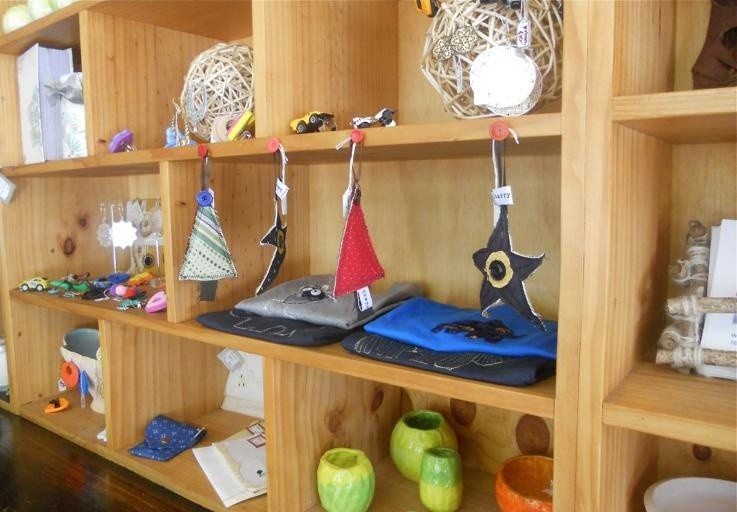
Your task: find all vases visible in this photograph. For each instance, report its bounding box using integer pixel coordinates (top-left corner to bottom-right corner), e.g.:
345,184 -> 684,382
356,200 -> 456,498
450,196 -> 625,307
316,447 -> 377,512
390,409 -> 459,484
417,447 -> 464,512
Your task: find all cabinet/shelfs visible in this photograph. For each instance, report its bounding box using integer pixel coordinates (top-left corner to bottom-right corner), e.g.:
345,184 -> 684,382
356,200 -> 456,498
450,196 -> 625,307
576,1 -> 736,512
1,0 -> 588,512
